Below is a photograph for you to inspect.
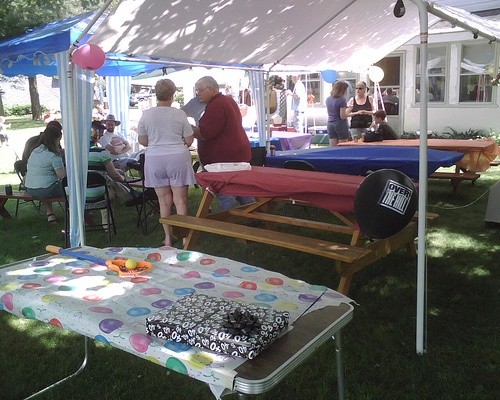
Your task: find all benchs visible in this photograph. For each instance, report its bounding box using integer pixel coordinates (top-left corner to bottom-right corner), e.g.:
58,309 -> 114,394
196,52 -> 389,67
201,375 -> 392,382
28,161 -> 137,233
0,189 -> 67,219
283,198 -> 441,222
158,212 -> 374,263
431,172 -> 481,181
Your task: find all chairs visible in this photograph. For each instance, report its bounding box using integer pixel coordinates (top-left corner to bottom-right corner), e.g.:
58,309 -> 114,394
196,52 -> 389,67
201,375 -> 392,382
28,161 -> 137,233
251,145 -> 267,167
125,153 -> 161,236
14,161 -> 65,218
283,161 -> 318,221
61,171 -> 117,248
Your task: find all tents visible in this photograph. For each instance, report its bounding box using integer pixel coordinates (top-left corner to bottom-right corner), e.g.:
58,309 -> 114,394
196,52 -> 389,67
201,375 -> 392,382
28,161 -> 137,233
0,9 -> 266,247
416,56 -> 488,102
65,0 -> 500,356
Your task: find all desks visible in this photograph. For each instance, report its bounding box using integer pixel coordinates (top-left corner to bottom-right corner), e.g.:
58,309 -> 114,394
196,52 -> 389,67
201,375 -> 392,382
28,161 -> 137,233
264,147 -> 463,180
338,139 -> 498,193
182,164 -> 420,295
0,246 -> 361,400
246,130 -> 311,150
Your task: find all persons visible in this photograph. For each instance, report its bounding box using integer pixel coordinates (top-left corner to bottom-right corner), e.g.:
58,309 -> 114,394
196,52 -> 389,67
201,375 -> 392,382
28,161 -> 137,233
0,74 -> 400,248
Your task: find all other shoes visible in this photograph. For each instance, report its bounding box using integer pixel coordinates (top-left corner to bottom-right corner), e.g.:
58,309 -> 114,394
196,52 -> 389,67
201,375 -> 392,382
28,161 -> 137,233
162,240 -> 172,246
125,197 -> 143,207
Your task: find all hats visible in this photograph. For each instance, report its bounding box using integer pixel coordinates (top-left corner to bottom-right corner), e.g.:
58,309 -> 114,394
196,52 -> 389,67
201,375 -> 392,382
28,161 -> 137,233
102,114 -> 121,125
92,120 -> 107,129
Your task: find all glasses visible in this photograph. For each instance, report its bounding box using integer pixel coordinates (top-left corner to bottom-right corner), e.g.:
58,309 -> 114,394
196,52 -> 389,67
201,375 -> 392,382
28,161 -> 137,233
195,86 -> 209,93
355,87 -> 364,90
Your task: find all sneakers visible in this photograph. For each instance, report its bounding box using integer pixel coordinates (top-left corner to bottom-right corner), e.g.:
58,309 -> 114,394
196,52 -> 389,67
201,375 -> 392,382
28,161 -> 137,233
47,213 -> 56,222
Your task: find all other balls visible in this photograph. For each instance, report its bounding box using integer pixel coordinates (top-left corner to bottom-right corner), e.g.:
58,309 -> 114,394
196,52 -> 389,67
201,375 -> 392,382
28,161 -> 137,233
125,259 -> 136,269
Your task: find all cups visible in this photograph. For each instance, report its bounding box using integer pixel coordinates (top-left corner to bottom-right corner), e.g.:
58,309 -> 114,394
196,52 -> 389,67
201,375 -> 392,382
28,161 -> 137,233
134,143 -> 139,152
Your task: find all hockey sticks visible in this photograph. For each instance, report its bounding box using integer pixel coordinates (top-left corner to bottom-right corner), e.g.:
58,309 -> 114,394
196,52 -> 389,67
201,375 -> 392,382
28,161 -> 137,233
45,245 -> 152,278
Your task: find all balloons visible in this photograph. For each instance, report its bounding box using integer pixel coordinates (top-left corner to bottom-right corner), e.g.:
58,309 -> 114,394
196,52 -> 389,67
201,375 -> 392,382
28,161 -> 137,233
72,49 -> 79,66
321,70 -> 338,83
369,66 -> 384,83
79,44 -> 105,70
